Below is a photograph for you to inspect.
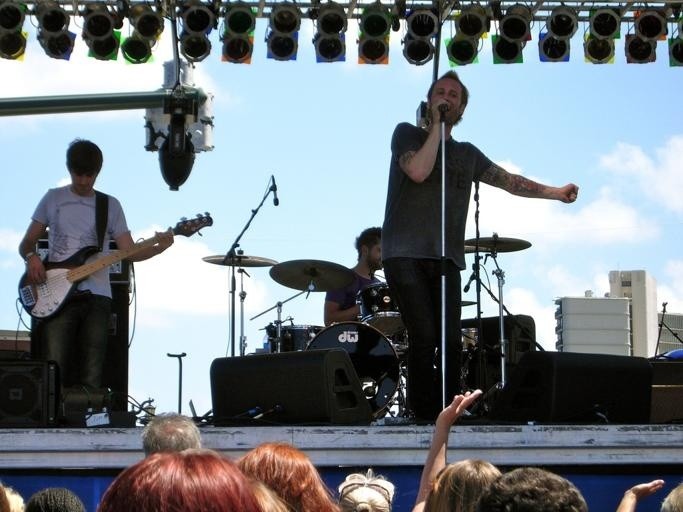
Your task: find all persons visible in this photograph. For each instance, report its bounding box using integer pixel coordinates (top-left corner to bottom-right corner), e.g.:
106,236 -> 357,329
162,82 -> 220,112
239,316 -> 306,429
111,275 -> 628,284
414,390 -> 502,511
475,468 -> 586,511
321,226 -> 383,328
17,134 -> 175,421
383,70 -> 580,426
618,479 -> 682,512
0,488 -> 86,512
98,411 -> 394,512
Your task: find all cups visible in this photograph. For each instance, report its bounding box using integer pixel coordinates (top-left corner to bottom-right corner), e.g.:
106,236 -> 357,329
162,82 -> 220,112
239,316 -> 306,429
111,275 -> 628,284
338,482 -> 392,512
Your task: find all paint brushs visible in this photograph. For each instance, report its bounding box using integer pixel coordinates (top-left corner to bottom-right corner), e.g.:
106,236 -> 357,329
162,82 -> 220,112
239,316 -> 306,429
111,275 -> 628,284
85,412 -> 111,427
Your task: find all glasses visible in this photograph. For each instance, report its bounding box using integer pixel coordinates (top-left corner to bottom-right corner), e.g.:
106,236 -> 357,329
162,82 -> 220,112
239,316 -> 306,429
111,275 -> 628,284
0,1 -> 683,67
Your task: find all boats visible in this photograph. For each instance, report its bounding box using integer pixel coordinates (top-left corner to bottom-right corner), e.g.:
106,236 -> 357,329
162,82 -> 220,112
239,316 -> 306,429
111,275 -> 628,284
30,283 -> 129,428
648,359 -> 683,424
461,314 -> 536,425
0,359 -> 62,429
210,346 -> 374,428
488,351 -> 655,425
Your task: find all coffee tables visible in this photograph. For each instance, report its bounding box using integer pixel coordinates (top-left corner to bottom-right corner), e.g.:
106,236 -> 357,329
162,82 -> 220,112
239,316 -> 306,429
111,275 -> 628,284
463,237 -> 531,253
459,299 -> 478,307
202,254 -> 278,267
268,259 -> 356,293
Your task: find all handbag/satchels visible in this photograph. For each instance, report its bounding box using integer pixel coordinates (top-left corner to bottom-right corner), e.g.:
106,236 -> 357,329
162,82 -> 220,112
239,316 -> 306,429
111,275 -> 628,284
22,252 -> 39,261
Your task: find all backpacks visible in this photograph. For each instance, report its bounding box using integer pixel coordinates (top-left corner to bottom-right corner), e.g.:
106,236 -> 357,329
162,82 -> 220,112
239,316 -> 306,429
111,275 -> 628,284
268,325 -> 325,352
461,327 -> 482,353
303,322 -> 403,420
356,282 -> 406,337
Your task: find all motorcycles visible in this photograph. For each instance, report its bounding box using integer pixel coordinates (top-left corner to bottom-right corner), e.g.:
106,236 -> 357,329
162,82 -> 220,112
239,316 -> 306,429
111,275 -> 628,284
469,268 -> 507,414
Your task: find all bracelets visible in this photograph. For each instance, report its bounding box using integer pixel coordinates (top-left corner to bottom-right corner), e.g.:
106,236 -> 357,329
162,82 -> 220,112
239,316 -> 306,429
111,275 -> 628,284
22,252 -> 39,261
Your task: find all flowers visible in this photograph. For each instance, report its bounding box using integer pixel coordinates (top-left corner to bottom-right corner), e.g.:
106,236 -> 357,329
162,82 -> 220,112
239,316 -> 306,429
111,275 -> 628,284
438,103 -> 450,112
463,272 -> 476,293
271,175 -> 279,206
